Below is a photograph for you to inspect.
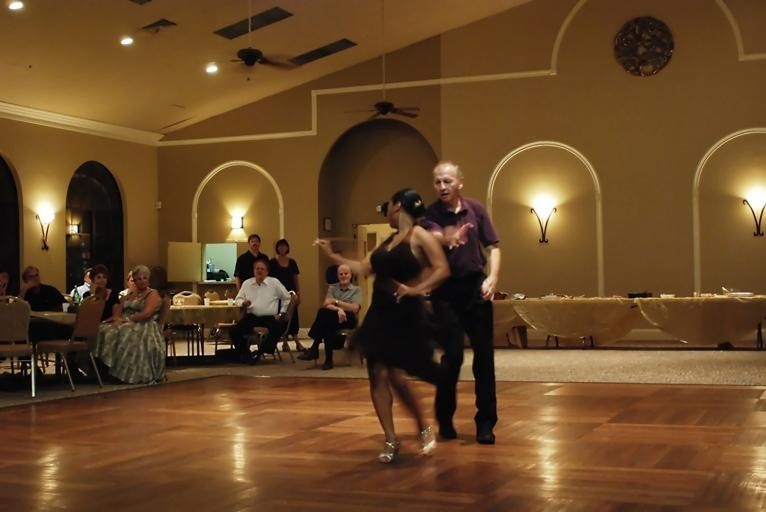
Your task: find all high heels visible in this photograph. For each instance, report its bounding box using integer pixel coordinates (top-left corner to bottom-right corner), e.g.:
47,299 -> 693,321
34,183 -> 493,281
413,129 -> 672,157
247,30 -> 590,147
377,440 -> 400,463
415,424 -> 436,457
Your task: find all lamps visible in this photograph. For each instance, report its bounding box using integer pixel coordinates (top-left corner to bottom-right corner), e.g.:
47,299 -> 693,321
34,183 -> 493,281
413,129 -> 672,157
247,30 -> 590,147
531,192 -> 556,243
36,198 -> 55,250
743,184 -> 766,236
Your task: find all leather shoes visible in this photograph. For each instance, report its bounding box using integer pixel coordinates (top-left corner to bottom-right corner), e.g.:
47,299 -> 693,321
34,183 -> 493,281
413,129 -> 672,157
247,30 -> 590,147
241,350 -> 260,365
477,430 -> 495,444
321,360 -> 332,369
297,348 -> 319,360
440,420 -> 456,439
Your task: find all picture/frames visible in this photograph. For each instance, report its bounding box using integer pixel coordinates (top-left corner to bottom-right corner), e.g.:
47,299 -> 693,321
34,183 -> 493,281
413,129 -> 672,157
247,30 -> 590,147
324,216 -> 333,231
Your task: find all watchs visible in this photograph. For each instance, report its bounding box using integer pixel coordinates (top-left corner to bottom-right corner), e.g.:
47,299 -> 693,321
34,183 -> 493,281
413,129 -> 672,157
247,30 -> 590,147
278,312 -> 284,317
334,298 -> 339,305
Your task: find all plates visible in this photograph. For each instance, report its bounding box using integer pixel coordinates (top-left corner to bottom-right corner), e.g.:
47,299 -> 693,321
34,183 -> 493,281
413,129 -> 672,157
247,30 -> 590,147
660,294 -> 675,298
728,292 -> 753,297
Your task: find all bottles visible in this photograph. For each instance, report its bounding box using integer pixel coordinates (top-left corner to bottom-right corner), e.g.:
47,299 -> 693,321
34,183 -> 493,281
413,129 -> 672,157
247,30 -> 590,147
72,285 -> 81,304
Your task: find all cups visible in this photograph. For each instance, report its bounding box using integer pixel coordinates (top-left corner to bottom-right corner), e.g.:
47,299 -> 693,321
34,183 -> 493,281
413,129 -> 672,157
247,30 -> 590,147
204,298 -> 210,307
62,302 -> 69,313
228,298 -> 233,306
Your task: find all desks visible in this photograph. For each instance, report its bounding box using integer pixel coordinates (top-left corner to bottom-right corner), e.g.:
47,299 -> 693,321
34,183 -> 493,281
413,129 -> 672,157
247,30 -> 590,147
488,294 -> 766,353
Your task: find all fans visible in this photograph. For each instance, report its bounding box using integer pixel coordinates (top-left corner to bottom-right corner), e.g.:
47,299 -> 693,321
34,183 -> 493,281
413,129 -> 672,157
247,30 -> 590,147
343,0 -> 420,124
216,0 -> 302,71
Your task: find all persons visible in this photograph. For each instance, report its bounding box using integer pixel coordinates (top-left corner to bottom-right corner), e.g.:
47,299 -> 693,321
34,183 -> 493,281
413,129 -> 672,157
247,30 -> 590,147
266,238 -> 307,352
232,233 -> 269,293
425,160 -> 501,445
0,263 -> 166,392
325,250 -> 359,284
295,263 -> 362,371
310,186 -> 451,463
227,258 -> 291,365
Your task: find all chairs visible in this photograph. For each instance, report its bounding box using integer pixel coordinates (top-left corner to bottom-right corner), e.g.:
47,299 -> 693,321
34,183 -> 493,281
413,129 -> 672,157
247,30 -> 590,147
0,289 -> 363,399
